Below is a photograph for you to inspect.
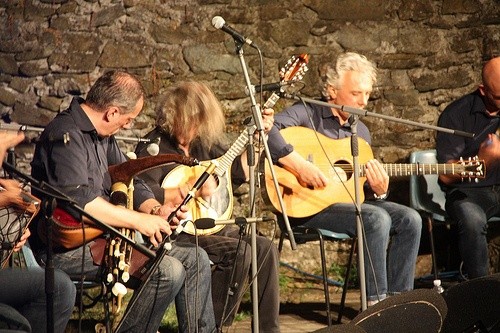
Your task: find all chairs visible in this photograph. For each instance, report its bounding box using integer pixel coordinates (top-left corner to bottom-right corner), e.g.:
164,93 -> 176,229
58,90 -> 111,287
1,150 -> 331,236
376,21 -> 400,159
278,218 -> 356,327
19,236 -> 110,333
410,148 -> 499,280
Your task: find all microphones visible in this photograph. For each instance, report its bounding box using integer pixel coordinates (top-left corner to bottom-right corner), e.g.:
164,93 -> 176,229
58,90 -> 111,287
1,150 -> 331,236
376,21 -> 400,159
212,16 -> 258,49
244,80 -> 297,96
126,152 -> 137,160
156,221 -> 187,257
195,217 -> 272,229
147,136 -> 160,156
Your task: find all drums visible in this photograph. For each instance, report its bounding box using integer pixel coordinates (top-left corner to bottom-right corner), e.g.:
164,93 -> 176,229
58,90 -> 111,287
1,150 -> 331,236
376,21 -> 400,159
0,183 -> 42,270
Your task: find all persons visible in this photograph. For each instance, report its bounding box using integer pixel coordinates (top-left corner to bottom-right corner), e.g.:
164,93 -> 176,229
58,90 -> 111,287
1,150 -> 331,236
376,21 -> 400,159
134,81 -> 282,333
436,55 -> 500,276
29,70 -> 217,333
266,52 -> 422,306
0,131 -> 78,333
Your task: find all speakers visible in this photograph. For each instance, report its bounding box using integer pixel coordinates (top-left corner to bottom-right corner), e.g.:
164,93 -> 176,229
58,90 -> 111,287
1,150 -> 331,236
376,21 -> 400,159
308,272 -> 500,333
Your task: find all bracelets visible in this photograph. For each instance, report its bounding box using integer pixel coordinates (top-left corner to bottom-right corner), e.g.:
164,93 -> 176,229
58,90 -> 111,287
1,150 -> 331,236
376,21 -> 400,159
151,206 -> 161,214
374,193 -> 388,200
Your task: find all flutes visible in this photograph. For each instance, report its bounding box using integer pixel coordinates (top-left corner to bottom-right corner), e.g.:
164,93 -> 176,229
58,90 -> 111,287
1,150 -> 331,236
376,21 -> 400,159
49,153 -> 278,333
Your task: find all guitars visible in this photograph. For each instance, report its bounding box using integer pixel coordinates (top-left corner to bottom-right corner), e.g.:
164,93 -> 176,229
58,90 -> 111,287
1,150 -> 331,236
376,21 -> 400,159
258,126 -> 487,223
